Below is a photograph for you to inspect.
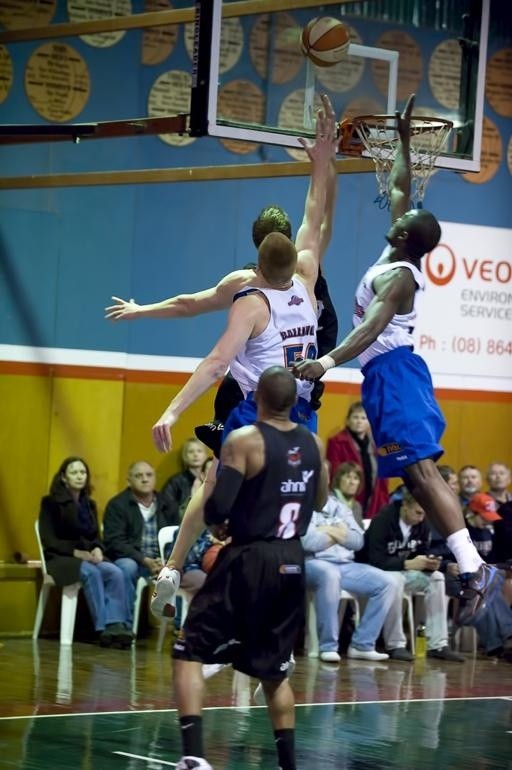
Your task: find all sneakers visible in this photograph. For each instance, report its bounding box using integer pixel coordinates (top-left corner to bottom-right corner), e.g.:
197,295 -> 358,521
150,567 -> 181,620
101,628 -> 133,649
253,657 -> 296,705
318,563 -> 507,662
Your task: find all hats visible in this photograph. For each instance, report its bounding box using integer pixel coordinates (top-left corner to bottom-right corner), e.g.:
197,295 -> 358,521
470,492 -> 502,521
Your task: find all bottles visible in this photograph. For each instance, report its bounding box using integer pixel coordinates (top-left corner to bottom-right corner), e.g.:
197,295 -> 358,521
414,620 -> 428,659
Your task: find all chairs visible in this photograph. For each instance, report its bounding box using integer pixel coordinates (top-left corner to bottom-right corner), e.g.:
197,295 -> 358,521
32,516 -> 449,656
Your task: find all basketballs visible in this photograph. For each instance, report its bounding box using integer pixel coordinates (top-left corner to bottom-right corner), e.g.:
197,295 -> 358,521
300,18 -> 347,69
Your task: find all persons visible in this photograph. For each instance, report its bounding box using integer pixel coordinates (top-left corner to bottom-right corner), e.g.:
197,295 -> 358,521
150,111 -> 341,704
300,402 -> 511,661
39,438 -> 231,647
170,362 -> 331,770
105,93 -> 340,459
297,93 -> 505,625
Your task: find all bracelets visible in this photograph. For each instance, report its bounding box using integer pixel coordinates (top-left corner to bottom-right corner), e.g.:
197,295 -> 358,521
317,354 -> 335,370
